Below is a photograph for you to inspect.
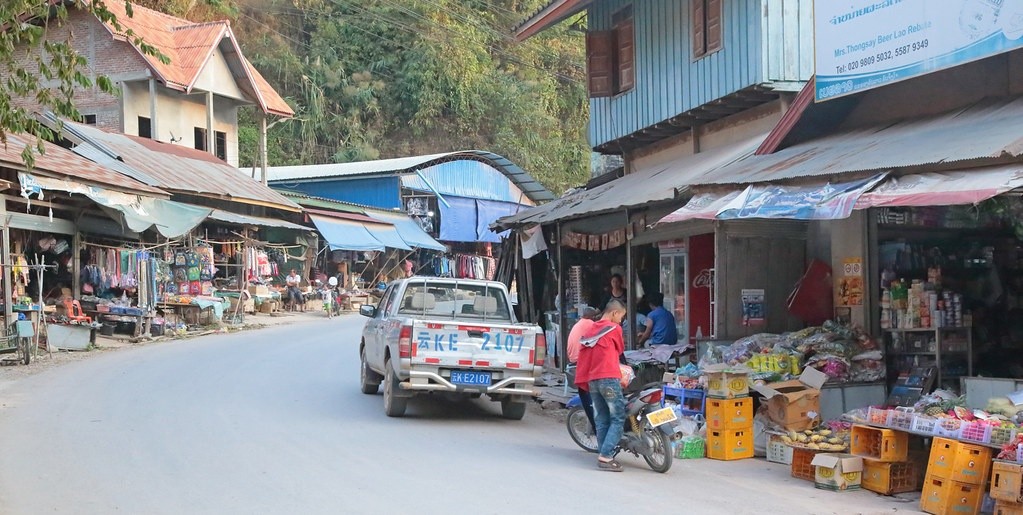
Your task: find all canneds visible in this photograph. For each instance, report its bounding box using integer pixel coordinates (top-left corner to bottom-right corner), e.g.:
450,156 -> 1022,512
897,308 -> 906,328
930,291 -> 963,327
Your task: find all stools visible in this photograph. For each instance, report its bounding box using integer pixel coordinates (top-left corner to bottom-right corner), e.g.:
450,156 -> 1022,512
563,362 -> 576,394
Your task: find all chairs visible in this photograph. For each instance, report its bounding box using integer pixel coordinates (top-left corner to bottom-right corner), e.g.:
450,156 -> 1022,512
462,304 -> 477,313
405,297 -> 423,310
63,298 -> 92,325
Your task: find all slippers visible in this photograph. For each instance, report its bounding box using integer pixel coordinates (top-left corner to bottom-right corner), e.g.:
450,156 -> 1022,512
596,459 -> 624,472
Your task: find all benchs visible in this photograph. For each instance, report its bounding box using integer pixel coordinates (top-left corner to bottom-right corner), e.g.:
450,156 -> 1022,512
410,292 -> 497,316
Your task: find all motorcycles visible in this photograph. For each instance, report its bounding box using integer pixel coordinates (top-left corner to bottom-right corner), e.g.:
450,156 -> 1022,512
564,363 -> 682,473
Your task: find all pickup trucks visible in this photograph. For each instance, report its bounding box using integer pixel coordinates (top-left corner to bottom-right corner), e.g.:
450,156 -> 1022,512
359,276 -> 545,420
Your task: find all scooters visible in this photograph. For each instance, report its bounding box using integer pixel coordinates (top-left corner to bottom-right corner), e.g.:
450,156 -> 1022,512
315,277 -> 341,316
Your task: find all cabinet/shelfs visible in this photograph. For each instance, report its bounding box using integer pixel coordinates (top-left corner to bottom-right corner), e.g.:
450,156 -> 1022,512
881,325 -> 972,392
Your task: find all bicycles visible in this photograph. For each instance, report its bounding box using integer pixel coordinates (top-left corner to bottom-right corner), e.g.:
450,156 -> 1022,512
9,319 -> 31,365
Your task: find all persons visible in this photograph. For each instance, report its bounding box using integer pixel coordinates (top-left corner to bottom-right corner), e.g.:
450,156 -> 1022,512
638,292 -> 676,349
592,273 -> 628,317
286,269 -> 306,313
567,307 -> 599,364
622,294 -> 652,334
321,283 -> 335,319
573,298 -> 627,471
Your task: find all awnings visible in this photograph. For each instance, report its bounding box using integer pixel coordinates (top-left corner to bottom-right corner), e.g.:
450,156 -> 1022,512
307,214 -> 386,253
433,195 -> 531,244
184,202 -> 317,233
365,211 -> 447,254
655,162 -> 1023,230
17,171 -> 214,240
362,221 -> 414,252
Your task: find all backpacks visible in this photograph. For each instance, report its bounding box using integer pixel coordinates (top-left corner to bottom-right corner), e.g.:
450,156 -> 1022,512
202,280 -> 213,296
174,268 -> 188,283
199,251 -> 211,265
175,252 -> 186,266
185,250 -> 199,267
179,282 -> 190,295
167,284 -> 178,295
199,266 -> 211,281
190,281 -> 201,295
186,267 -> 200,281
162,271 -> 175,284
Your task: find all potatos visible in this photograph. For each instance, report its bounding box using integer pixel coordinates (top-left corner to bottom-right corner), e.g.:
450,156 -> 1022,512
780,430 -> 848,449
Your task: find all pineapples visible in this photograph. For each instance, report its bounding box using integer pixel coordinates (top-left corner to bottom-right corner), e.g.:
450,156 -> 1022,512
926,397 -> 965,415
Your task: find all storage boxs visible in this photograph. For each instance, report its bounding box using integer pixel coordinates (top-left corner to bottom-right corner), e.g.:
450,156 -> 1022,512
248,285 -> 268,295
662,361 -> 1023,515
81,301 -> 164,337
261,301 -> 274,312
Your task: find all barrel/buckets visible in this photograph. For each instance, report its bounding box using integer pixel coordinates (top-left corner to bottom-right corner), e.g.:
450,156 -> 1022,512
101,321 -> 117,336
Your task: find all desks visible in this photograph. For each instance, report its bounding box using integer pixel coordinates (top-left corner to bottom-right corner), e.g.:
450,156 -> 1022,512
343,295 -> 369,310
158,294 -> 281,324
84,309 -> 157,339
